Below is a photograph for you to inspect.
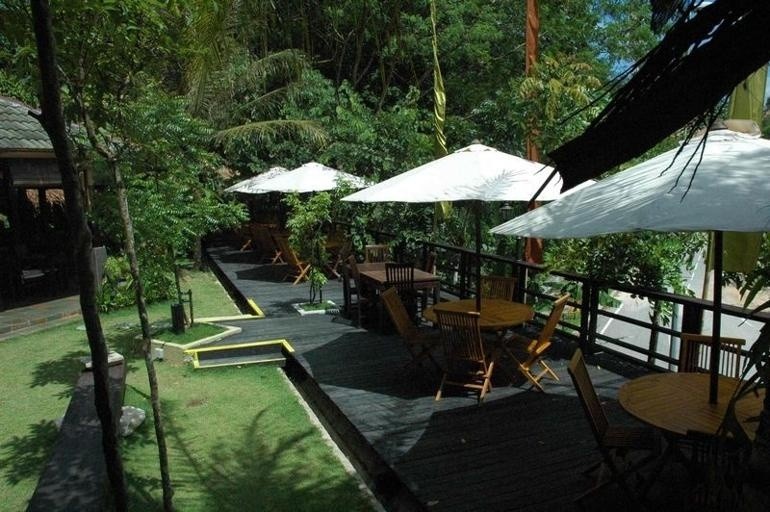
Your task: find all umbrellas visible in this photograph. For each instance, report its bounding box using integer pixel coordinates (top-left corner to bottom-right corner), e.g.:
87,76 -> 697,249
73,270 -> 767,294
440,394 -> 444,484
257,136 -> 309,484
339,137 -> 600,313
253,159 -> 377,203
487,121 -> 770,404
223,164 -> 291,224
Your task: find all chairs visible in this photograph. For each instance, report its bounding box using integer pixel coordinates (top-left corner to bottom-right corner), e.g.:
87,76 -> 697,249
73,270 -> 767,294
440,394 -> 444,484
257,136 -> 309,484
340,244 -> 443,330
567,334 -> 768,511
380,274 -> 571,403
241,222 -> 353,287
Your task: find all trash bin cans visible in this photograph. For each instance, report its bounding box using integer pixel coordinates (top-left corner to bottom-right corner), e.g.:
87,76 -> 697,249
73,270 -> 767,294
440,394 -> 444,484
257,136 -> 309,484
172,304 -> 184,331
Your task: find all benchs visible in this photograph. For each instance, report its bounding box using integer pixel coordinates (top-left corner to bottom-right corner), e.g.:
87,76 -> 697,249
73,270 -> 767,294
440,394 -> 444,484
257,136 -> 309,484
26,360 -> 127,512
